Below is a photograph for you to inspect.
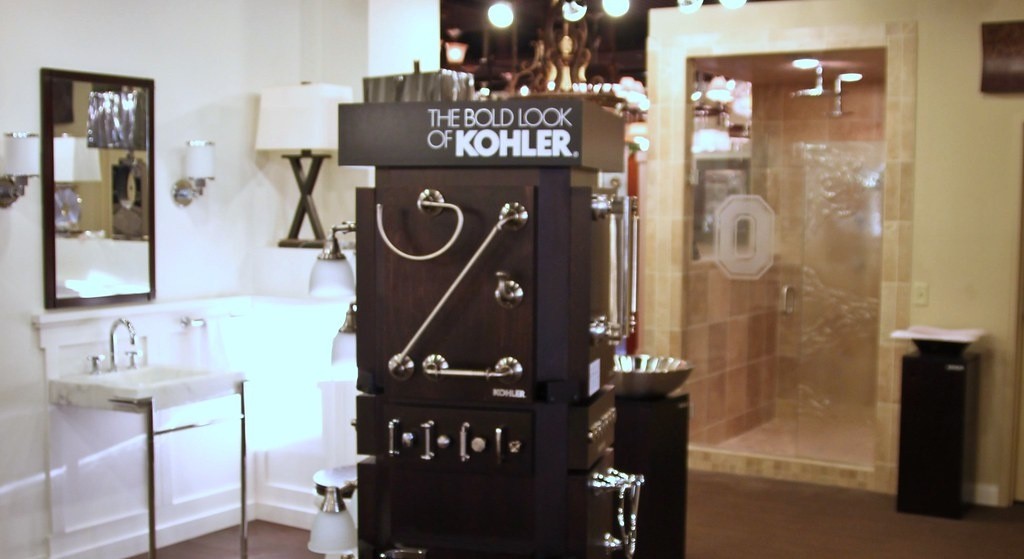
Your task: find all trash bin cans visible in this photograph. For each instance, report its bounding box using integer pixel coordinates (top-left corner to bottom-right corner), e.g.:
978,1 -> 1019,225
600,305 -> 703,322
613,354 -> 697,559
890,325 -> 989,519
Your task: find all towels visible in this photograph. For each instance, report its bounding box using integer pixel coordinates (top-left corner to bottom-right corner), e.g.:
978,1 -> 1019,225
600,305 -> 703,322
190,310 -> 236,420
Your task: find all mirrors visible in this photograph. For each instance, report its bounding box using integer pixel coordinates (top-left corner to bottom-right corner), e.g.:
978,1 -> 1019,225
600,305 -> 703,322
39,66 -> 157,310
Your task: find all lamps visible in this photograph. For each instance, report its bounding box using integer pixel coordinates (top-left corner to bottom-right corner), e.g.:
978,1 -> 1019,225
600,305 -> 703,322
0,131 -> 41,209
308,221 -> 356,298
171,139 -> 216,208
330,301 -> 358,365
255,81 -> 354,249
53,131 -> 101,239
311,474 -> 359,555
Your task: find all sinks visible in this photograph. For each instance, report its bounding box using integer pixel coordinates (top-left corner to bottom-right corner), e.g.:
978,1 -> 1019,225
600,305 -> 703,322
47,364 -> 247,415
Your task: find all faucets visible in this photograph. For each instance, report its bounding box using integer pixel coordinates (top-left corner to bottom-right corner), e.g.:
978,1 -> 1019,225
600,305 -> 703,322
105,317 -> 138,374
